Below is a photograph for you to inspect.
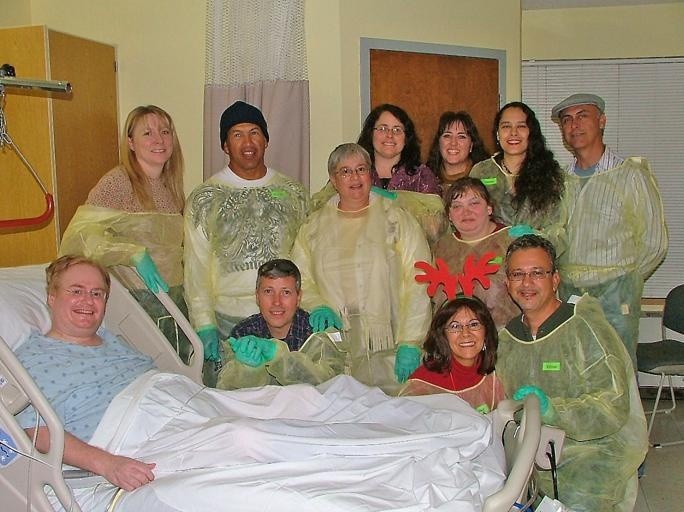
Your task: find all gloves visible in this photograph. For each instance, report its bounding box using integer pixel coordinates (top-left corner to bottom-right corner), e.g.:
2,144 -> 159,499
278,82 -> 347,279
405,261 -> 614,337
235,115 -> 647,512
309,305 -> 342,331
509,224 -> 533,237
513,385 -> 549,415
237,336 -> 277,363
370,185 -> 397,202
227,336 -> 263,367
194,324 -> 218,360
131,249 -> 169,294
394,346 -> 424,383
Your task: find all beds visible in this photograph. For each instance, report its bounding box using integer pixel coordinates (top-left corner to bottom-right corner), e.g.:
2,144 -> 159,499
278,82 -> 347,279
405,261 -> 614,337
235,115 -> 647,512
0,255 -> 542,512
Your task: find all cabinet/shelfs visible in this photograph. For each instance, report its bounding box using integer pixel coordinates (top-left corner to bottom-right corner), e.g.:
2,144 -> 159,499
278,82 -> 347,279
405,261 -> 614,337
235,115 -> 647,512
0,24 -> 134,270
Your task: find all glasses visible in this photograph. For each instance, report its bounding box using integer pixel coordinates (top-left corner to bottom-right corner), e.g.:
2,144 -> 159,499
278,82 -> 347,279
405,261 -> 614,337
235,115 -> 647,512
373,125 -> 406,135
54,285 -> 108,301
335,166 -> 371,177
508,268 -> 554,281
442,321 -> 486,331
260,259 -> 295,276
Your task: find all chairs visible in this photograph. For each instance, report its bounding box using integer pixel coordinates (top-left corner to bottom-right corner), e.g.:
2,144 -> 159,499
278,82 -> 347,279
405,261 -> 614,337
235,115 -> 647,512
635,285 -> 684,451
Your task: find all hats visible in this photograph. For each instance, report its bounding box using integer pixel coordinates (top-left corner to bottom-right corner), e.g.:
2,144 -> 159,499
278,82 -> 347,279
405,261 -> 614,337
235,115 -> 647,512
550,94 -> 605,123
219,101 -> 270,151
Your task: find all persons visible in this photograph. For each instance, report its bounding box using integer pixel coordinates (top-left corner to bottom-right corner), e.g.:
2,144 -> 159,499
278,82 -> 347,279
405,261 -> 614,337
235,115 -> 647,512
83,105 -> 186,364
356,104 -> 438,195
427,110 -> 485,190
5,254 -> 164,494
469,102 -> 573,250
291,143 -> 432,382
429,174 -> 520,325
493,235 -> 648,512
181,100 -> 308,362
398,292 -> 507,424
551,94 -> 669,370
218,259 -> 347,388
